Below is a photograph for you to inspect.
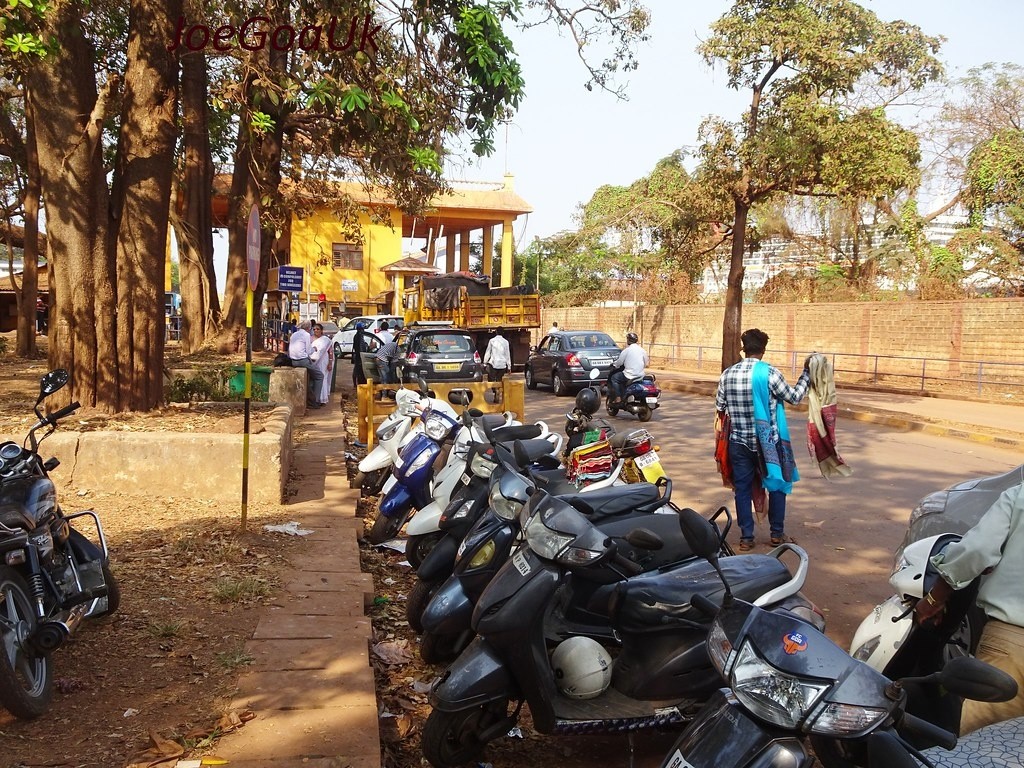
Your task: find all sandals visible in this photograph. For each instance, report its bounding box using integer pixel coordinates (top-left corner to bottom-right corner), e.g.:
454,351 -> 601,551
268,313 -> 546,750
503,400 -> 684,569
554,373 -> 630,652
770,534 -> 798,547
741,540 -> 756,551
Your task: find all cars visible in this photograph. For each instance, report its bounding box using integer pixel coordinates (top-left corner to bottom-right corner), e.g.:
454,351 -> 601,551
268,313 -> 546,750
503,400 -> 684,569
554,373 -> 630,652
526,330 -> 623,397
888,463 -> 1024,573
305,320 -> 341,339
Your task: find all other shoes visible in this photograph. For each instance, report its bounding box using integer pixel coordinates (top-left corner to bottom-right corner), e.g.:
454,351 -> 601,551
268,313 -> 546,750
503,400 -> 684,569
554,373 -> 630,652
308,400 -> 320,409
382,397 -> 394,402
610,400 -> 621,407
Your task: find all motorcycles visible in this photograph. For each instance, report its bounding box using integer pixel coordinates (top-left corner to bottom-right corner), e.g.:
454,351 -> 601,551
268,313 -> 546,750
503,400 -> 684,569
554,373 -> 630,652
562,368 -> 667,485
1,369 -> 121,719
605,351 -> 661,421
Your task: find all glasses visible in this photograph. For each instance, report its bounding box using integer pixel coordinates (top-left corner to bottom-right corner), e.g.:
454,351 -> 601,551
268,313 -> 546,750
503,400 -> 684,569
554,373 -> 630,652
314,328 -> 323,331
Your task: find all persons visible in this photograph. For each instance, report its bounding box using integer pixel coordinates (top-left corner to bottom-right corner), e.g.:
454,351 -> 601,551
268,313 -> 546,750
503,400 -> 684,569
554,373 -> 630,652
547,322 -> 560,345
165,302 -> 182,340
483,326 -> 511,403
352,321 -> 368,387
378,309 -> 385,321
915,481 -> 1024,737
610,333 -> 649,403
338,313 -> 352,329
36,293 -> 44,307
370,321 -> 402,352
715,328 -> 817,549
283,313 -> 335,409
327,312 -> 334,322
374,342 -> 401,402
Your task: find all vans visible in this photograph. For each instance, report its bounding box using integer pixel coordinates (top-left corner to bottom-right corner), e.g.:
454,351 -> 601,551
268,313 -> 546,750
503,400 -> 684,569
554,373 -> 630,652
332,312 -> 405,359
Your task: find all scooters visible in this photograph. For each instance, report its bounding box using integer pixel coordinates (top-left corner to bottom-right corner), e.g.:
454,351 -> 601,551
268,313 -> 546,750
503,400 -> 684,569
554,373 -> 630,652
357,374 -> 744,689
415,440 -> 829,768
846,525 -> 982,683
652,509 -> 1023,766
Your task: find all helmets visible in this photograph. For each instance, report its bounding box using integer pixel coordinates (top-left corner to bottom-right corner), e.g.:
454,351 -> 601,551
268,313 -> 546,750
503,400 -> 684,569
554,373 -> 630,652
576,389 -> 600,414
626,332 -> 638,344
550,636 -> 613,702
357,323 -> 367,329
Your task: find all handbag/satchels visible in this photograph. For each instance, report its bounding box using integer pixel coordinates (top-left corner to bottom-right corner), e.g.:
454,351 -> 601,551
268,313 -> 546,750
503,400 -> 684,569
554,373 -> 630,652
274,353 -> 291,367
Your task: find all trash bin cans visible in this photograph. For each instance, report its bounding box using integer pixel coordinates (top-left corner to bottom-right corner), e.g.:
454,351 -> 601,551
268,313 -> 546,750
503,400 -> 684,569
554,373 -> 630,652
229,366 -> 275,402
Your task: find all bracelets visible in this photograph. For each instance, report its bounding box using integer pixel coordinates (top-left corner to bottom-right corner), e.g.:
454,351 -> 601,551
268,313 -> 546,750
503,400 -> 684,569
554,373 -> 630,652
329,359 -> 333,361
330,353 -> 333,354
927,587 -> 936,605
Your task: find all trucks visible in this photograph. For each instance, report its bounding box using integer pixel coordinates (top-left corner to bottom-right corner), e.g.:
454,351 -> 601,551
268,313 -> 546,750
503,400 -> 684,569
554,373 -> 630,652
401,275 -> 542,366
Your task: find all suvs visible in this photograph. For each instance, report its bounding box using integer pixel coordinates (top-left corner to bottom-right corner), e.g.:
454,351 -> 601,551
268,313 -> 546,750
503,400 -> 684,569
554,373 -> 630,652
361,321 -> 485,383
